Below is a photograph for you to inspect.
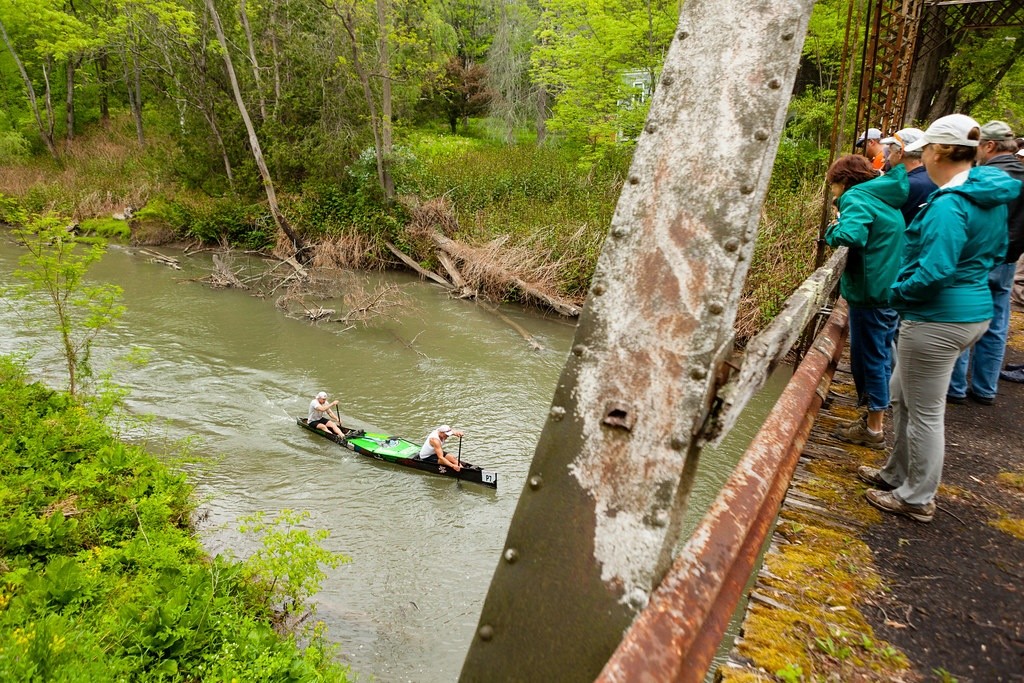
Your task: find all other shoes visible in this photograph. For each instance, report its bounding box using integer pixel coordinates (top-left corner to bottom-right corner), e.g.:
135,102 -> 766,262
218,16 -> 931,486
947,395 -> 967,404
966,387 -> 993,405
1000,363 -> 1024,382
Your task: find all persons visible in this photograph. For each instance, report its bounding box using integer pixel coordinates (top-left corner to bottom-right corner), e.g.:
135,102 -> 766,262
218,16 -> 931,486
419,424 -> 464,473
307,392 -> 344,438
859,112 -> 1022,523
858,121 -> 1024,406
826,153 -> 911,450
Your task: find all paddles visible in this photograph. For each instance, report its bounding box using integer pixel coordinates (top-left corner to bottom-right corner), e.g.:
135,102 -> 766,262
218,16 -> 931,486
457,436 -> 462,482
388,435 -> 422,447
336,404 -> 341,427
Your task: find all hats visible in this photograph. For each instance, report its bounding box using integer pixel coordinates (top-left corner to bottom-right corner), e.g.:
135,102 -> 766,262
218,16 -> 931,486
980,121 -> 1014,140
879,128 -> 925,151
439,425 -> 453,436
855,128 -> 882,148
316,392 -> 327,399
1015,149 -> 1024,157
904,114 -> 981,152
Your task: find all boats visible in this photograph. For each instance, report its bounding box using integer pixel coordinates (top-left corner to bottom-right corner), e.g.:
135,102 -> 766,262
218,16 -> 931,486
296,415 -> 498,490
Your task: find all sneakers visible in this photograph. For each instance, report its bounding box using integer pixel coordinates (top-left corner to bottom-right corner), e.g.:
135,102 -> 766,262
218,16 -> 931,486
829,382 -> 858,400
857,466 -> 896,489
866,489 -> 936,522
834,411 -> 886,449
